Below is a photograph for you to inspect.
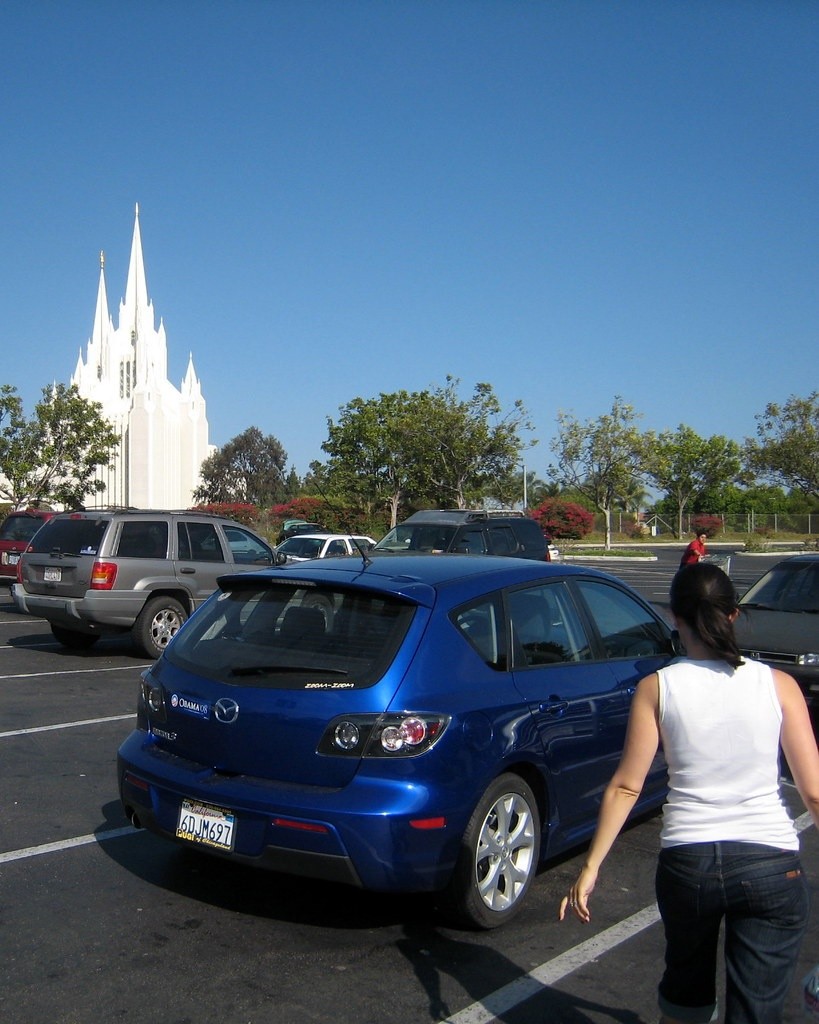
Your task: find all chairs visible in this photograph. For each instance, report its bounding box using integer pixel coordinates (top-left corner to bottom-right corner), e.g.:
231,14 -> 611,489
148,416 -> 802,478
510,593 -> 563,665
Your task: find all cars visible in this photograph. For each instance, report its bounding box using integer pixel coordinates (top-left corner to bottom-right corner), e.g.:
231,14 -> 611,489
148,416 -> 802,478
275,519 -> 327,546
273,534 -> 378,562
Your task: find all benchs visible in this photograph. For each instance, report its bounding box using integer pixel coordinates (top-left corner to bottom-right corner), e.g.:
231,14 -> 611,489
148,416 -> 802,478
246,602 -> 388,657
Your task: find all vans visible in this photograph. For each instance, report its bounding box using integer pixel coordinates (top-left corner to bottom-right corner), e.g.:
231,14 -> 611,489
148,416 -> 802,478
731,550 -> 819,727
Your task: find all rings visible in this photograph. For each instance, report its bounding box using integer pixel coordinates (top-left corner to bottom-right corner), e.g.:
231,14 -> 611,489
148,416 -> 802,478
570,901 -> 577,908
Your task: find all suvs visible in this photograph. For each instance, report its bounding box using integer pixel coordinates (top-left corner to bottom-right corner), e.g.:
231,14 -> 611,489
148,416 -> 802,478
11,505 -> 336,662
0,510 -> 70,583
116,551 -> 693,930
372,508 -> 552,564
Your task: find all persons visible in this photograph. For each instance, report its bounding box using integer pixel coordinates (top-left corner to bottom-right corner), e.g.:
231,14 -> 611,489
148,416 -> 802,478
679,531 -> 709,567
557,562 -> 819,1024
